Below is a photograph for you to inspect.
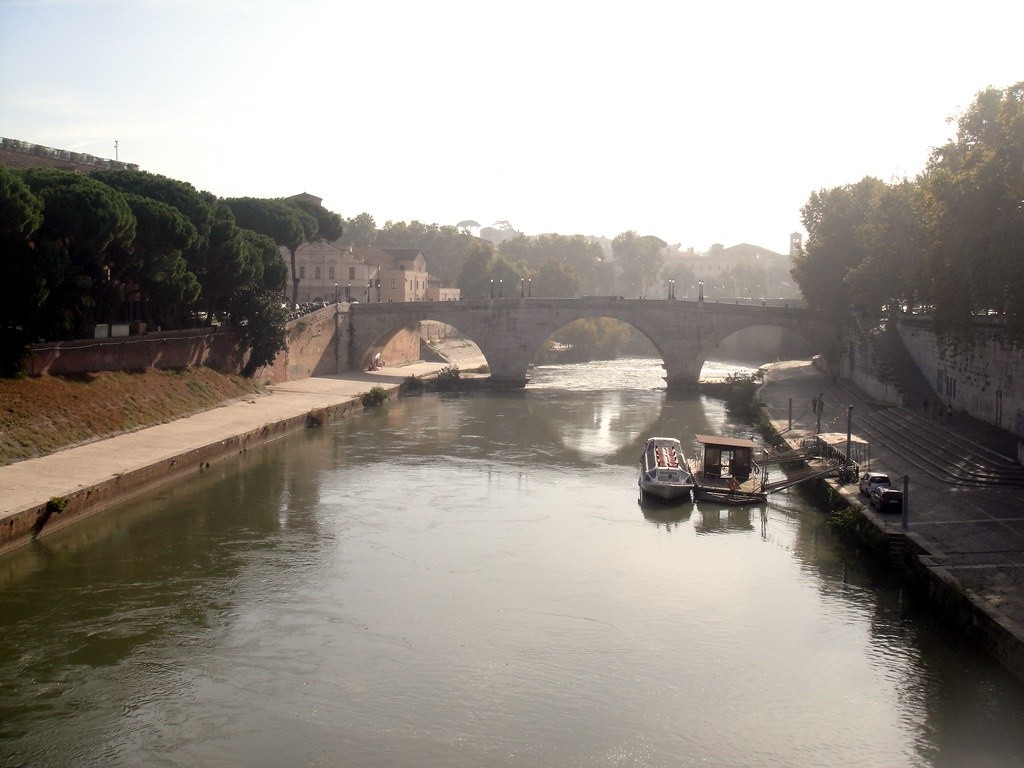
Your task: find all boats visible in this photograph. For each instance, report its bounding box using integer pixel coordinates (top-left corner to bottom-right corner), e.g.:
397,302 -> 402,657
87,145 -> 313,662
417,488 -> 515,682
686,434 -> 769,506
638,436 -> 694,501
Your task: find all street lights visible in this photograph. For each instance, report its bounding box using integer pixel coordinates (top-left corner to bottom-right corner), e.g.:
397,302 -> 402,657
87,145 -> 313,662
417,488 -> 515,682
521,277 -> 524,297
528,277 -> 532,297
377,284 -> 380,302
500,279 -> 502,298
702,280 -> 704,301
491,279 -> 494,299
667,278 -> 672,299
672,279 -> 676,300
699,280 -> 701,301
368,283 -> 370,302
334,282 -> 338,303
348,283 -> 350,301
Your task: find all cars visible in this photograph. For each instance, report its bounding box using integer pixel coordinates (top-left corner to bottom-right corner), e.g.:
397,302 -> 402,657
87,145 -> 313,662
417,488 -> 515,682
859,472 -> 891,497
871,485 -> 902,513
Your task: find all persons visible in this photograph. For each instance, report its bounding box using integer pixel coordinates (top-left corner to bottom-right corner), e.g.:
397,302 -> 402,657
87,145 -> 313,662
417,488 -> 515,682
812,397 -> 817,413
923,398 -> 952,425
